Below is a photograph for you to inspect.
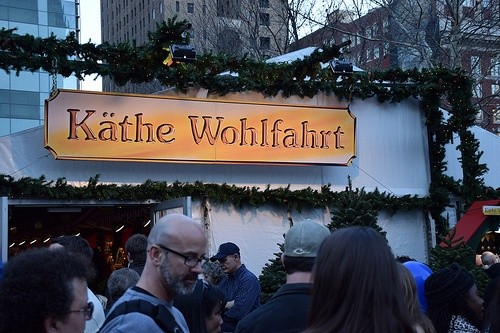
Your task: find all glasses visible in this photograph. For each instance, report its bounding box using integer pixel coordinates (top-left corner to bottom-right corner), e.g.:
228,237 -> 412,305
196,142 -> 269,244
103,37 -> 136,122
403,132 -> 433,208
44,302 -> 94,318
148,244 -> 209,267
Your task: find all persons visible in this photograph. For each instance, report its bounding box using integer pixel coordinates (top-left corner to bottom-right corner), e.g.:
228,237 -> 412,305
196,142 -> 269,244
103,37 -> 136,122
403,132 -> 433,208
0,214 -> 499,333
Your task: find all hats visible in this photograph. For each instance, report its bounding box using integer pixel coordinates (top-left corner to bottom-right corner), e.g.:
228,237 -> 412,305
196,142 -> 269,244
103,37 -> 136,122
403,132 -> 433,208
210,242 -> 240,262
284,219 -> 331,257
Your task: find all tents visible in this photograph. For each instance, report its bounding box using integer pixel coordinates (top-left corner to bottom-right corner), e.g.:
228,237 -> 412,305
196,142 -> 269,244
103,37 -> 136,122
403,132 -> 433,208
440,200 -> 500,249
